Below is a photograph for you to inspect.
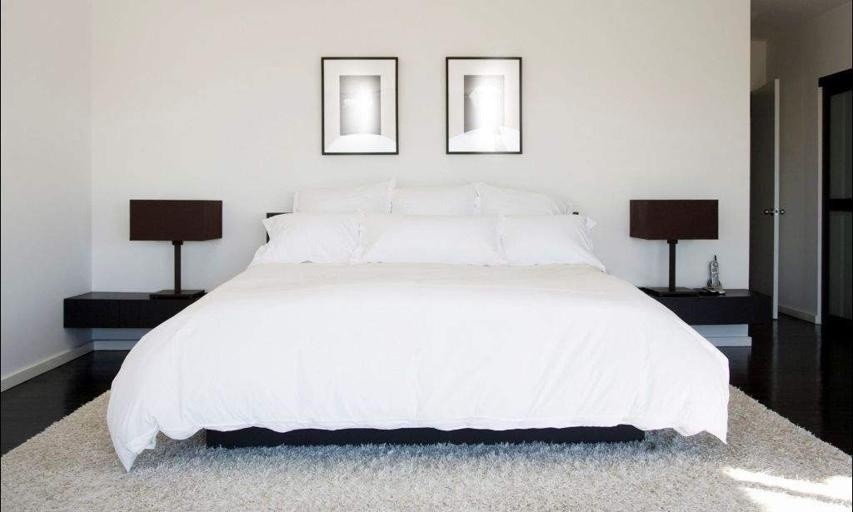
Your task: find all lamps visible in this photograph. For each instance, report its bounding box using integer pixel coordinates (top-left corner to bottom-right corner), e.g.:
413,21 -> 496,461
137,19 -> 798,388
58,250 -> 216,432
127,199 -> 222,298
627,198 -> 719,296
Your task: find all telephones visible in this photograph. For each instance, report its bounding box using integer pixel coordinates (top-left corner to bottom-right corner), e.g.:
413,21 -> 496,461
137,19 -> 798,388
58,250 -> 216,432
703,255 -> 726,294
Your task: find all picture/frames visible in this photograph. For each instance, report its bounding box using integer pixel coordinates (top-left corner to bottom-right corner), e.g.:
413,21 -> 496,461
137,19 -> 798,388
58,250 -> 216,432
320,56 -> 399,155
444,56 -> 522,155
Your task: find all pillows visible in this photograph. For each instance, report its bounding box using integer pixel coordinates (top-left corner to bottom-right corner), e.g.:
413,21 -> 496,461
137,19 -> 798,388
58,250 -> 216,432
493,213 -> 606,274
245,208 -> 361,264
473,186 -> 569,221
353,208 -> 497,269
388,182 -> 479,217
292,178 -> 398,213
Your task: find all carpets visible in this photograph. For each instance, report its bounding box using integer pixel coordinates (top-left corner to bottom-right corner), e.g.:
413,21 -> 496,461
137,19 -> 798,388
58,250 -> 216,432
0,386 -> 852,511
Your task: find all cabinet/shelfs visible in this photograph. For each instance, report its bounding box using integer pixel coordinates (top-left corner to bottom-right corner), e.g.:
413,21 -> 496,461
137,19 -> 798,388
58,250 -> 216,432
61,291 -> 208,328
640,286 -> 770,325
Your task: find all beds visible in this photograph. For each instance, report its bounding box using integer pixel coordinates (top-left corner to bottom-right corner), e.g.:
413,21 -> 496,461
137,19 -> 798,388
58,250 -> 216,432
104,212 -> 732,474
